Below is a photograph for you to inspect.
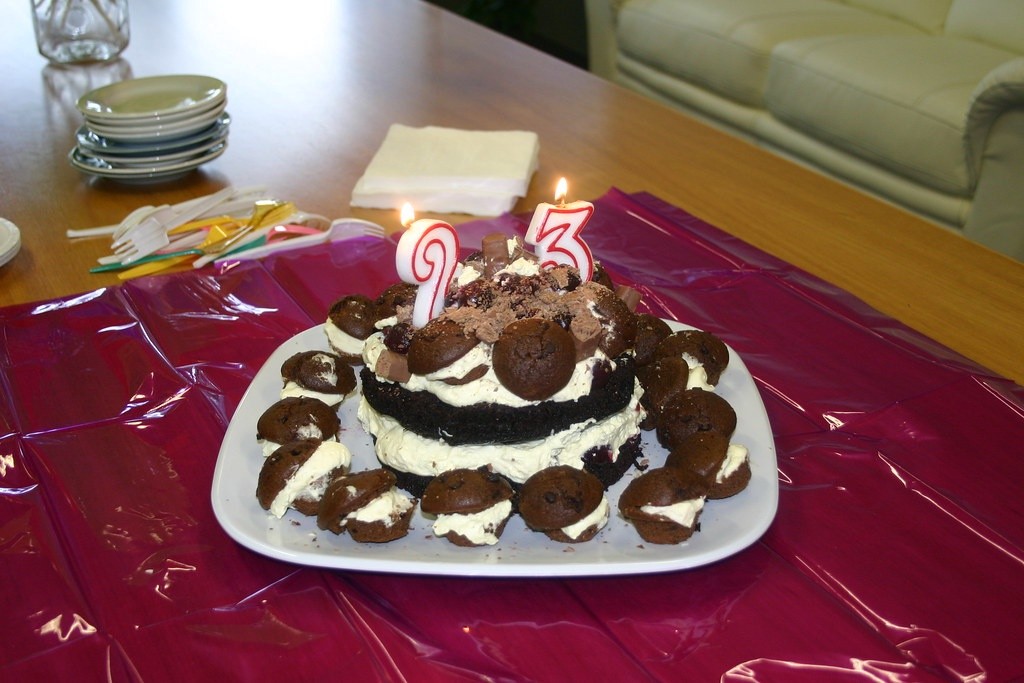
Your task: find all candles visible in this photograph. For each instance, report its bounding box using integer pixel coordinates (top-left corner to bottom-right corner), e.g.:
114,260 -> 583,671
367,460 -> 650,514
394,202 -> 459,329
525,177 -> 595,284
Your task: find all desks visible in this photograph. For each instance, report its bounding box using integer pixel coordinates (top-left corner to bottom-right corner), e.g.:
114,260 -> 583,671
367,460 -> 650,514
0,0 -> 1023,681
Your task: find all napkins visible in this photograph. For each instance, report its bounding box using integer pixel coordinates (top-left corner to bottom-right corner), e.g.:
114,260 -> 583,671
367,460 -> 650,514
348,123 -> 540,217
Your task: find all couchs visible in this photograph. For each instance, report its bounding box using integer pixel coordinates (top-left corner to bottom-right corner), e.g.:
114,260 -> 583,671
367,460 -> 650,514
585,0 -> 1023,263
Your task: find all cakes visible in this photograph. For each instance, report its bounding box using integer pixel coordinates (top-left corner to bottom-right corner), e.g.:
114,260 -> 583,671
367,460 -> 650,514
255,235 -> 752,546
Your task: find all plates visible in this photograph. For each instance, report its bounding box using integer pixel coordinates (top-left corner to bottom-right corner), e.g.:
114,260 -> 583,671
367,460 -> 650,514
0,217 -> 22,268
68,75 -> 233,184
210,318 -> 780,577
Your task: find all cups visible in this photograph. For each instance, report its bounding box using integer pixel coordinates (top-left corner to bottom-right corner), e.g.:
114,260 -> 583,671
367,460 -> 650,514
29,0 -> 131,66
